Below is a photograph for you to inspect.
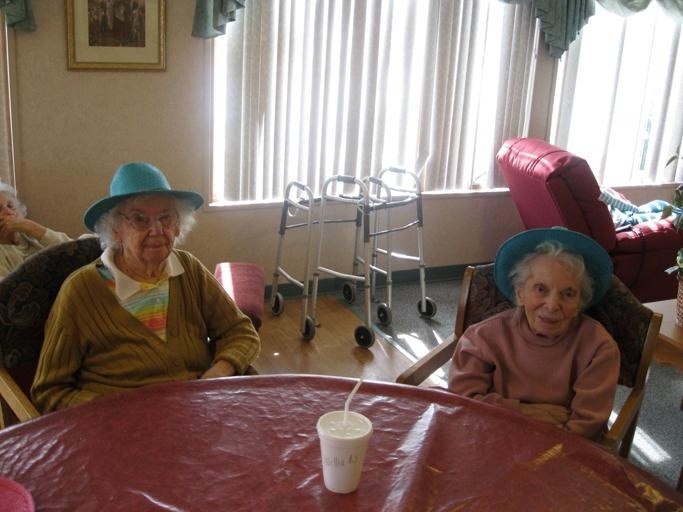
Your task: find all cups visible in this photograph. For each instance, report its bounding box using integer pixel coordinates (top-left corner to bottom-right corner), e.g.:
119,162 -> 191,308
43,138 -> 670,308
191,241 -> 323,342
314,410 -> 372,494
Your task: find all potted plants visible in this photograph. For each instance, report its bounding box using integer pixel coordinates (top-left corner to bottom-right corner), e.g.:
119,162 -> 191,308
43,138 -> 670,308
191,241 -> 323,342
666,152 -> 683,329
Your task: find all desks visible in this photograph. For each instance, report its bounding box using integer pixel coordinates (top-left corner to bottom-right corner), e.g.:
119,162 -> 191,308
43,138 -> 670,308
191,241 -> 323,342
643,298 -> 683,493
1,375 -> 683,512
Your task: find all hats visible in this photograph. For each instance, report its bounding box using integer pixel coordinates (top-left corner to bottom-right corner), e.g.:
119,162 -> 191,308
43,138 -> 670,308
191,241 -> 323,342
81,163 -> 204,232
494,222 -> 611,314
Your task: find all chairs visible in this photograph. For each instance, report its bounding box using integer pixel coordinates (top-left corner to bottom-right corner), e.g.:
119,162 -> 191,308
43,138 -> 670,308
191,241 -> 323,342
395,263 -> 664,457
0,238 -> 258,428
495,138 -> 683,304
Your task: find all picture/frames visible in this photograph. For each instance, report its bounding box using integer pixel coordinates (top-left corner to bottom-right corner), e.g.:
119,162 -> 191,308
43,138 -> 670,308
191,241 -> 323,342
65,0 -> 167,71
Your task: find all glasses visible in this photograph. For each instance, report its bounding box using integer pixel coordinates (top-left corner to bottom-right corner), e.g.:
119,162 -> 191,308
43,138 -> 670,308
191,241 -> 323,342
113,207 -> 181,230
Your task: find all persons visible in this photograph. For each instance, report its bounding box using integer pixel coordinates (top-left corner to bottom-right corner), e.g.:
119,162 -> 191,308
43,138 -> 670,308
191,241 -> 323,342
29,163 -> 262,411
0,179 -> 70,281
446,224 -> 622,443
